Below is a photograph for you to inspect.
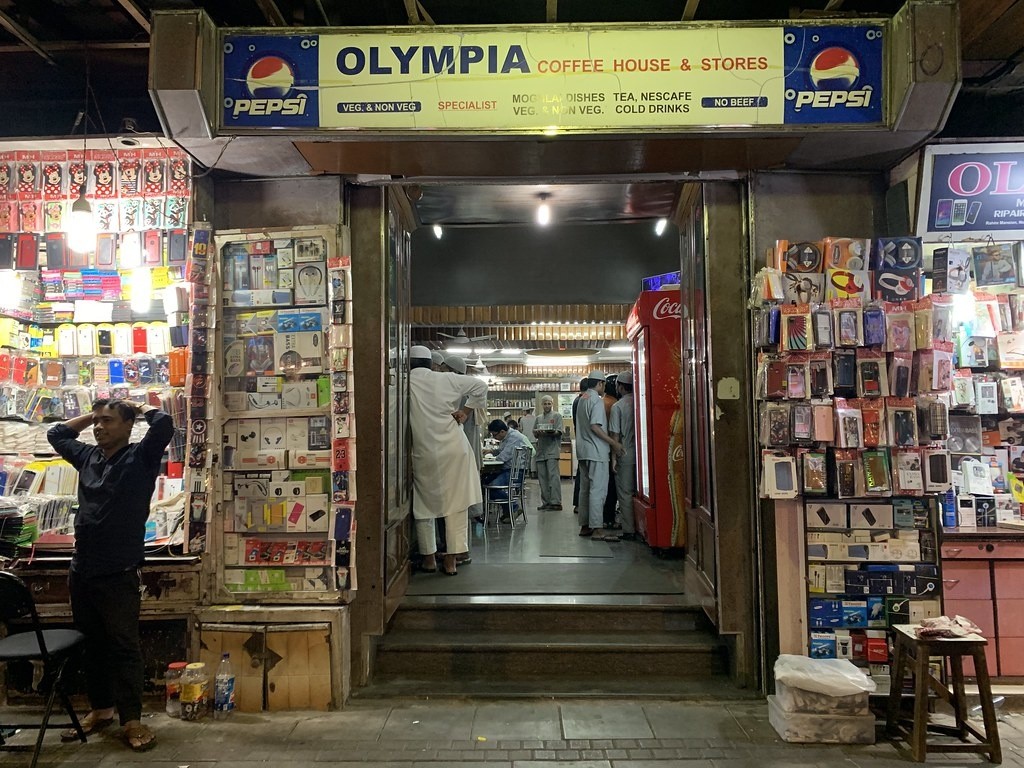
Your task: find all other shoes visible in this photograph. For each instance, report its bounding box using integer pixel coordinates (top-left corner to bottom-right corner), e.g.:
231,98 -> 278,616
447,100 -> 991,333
622,532 -> 637,541
537,502 -> 563,511
603,520 -> 623,529
574,505 -> 579,514
500,506 -> 523,523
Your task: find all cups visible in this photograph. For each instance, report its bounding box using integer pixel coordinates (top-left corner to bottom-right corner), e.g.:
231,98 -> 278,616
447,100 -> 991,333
536,423 -> 555,430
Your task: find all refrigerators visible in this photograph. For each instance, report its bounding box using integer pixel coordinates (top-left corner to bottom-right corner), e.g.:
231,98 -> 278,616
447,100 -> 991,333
625,289 -> 688,559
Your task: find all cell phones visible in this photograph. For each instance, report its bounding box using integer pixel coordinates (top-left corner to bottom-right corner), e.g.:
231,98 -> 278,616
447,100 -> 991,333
935,199 -> 982,228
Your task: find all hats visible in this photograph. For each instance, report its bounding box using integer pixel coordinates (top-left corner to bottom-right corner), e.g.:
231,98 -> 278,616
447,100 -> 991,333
390,346 -> 397,359
410,345 -> 432,360
504,411 -> 511,417
432,351 -> 445,366
588,370 -> 606,382
617,371 -> 632,384
443,355 -> 466,374
522,406 -> 530,410
541,395 -> 553,404
605,374 -> 618,380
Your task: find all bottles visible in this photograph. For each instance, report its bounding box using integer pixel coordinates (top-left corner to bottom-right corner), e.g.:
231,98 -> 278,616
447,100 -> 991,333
165,662 -> 187,717
408,303 -> 636,341
213,653 -> 236,721
180,662 -> 209,721
465,363 -> 632,407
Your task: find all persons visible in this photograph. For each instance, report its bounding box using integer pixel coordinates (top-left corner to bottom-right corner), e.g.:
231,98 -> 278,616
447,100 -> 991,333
409,346 -> 488,576
483,419 -> 528,522
508,420 -> 537,457
519,407 -> 536,441
532,395 -> 563,511
503,411 -> 512,425
48,396 -> 175,752
571,369 -> 637,543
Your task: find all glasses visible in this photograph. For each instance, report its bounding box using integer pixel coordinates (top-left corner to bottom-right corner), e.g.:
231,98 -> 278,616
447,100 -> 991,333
492,432 -> 501,438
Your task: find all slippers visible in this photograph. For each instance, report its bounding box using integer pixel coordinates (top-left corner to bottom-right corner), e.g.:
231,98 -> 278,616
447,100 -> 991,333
61,712 -> 115,740
591,534 -> 621,542
578,525 -> 592,536
415,562 -> 437,573
439,566 -> 458,577
119,726 -> 158,752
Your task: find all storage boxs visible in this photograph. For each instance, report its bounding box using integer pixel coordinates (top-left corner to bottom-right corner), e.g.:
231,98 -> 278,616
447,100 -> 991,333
220,237 -> 332,591
956,332 -> 1024,529
930,247 -> 971,295
767,498 -> 940,745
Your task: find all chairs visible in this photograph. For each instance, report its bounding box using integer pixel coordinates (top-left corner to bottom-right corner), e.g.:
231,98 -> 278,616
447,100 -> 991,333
0,570 -> 84,768
482,445 -> 534,530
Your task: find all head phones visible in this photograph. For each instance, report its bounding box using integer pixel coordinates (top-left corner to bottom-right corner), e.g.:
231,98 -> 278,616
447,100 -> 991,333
831,271 -> 864,294
298,265 -> 322,296
879,274 -> 914,295
794,278 -> 812,305
223,340 -> 245,375
828,238 -> 864,270
884,238 -> 920,269
785,243 -> 821,273
247,338 -> 275,370
263,427 -> 284,446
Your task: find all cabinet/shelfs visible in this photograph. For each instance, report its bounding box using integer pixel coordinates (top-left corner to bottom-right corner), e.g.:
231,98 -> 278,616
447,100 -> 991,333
937,525 -> 1024,686
558,440 -> 573,480
0,563 -> 203,715
190,223 -> 358,605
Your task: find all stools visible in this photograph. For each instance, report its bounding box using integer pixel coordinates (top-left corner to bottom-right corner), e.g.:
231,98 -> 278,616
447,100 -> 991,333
884,624 -> 1005,765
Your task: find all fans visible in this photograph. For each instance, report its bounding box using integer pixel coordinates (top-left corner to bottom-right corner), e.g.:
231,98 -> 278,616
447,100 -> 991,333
466,355 -> 497,369
436,323 -> 497,344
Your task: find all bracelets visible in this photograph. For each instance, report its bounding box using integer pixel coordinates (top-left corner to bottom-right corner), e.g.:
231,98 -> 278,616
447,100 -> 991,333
136,402 -> 146,409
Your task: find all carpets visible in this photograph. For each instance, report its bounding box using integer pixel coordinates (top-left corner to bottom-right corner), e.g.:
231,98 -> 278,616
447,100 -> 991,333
402,561 -> 686,597
